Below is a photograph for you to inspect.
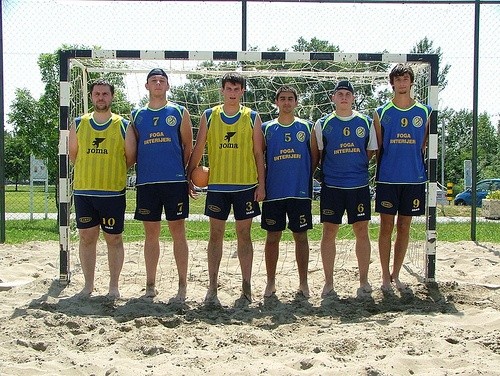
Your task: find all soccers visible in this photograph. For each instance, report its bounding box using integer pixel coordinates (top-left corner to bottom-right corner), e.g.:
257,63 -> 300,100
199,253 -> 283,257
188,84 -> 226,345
189,166 -> 209,188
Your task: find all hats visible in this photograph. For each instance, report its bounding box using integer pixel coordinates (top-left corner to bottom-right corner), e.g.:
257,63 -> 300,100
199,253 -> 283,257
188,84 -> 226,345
147,68 -> 168,78
334,80 -> 355,94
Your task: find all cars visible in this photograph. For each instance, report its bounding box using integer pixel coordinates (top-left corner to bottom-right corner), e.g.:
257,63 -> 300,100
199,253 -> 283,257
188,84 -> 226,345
126,176 -> 135,187
313,178 -> 321,201
454,178 -> 500,208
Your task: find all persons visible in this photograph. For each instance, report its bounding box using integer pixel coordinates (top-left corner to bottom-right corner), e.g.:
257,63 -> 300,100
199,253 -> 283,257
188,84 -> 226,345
129,68 -> 193,298
69,80 -> 137,298
187,72 -> 266,302
260,85 -> 320,297
373,64 -> 433,291
314,81 -> 378,296
